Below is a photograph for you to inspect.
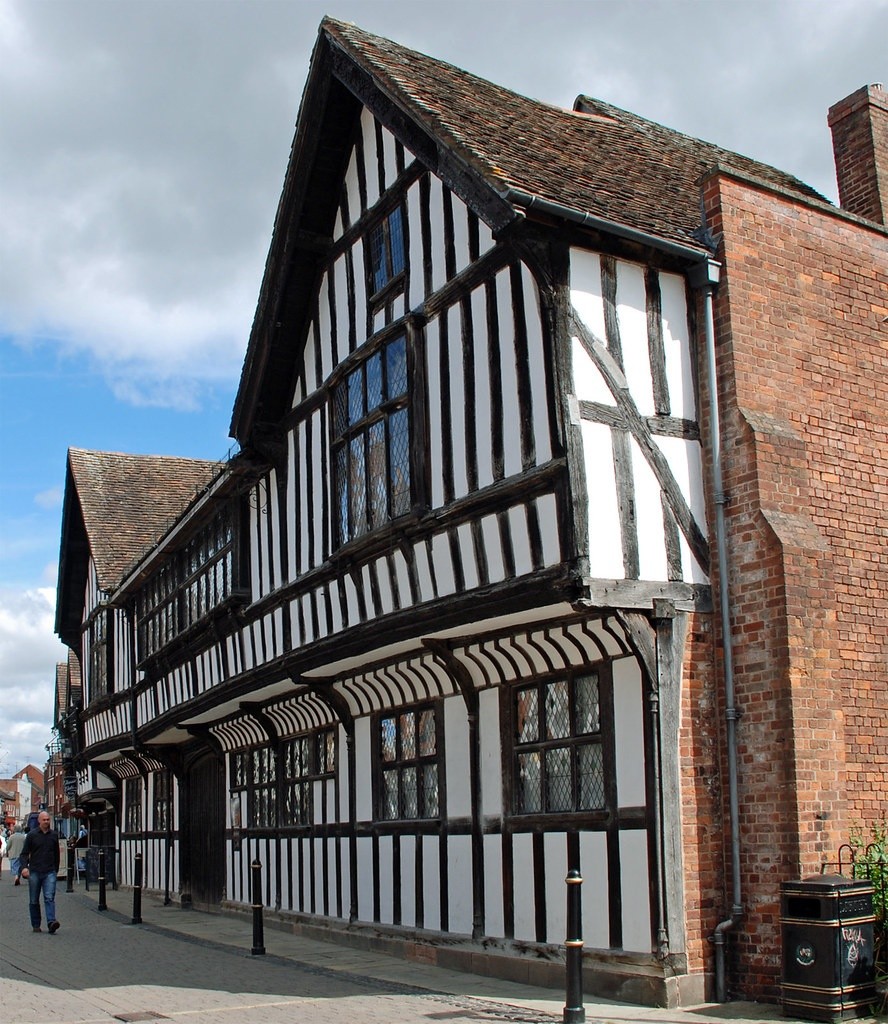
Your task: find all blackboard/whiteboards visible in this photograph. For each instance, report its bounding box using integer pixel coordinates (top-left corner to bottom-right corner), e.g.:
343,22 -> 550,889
85,849 -> 115,883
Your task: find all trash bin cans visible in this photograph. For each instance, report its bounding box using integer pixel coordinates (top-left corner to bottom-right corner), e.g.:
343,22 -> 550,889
778,874 -> 876,1024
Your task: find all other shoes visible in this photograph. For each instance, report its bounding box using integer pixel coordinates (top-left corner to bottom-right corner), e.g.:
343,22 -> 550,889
32,927 -> 42,933
14,875 -> 21,886
49,921 -> 61,934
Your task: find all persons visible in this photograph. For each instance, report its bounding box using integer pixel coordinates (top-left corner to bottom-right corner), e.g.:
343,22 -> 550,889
6,825 -> 26,886
79,825 -> 87,838
0,824 -> 30,879
18,811 -> 60,933
